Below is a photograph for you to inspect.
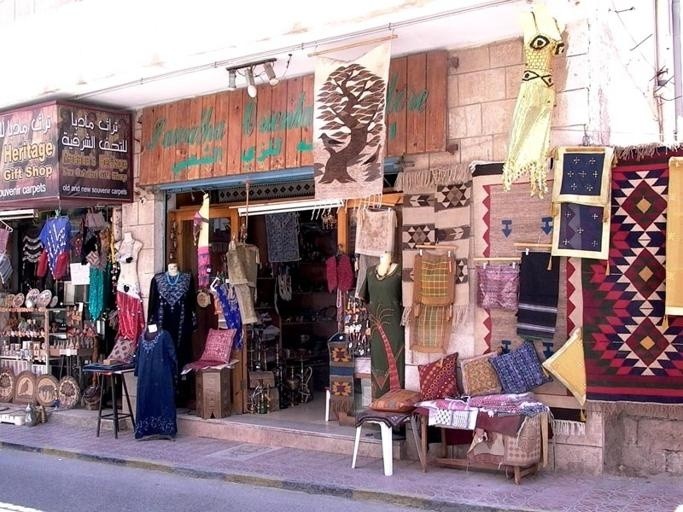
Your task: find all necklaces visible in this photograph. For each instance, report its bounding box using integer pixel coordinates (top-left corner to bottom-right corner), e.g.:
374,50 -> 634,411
164,271 -> 179,286
374,262 -> 392,281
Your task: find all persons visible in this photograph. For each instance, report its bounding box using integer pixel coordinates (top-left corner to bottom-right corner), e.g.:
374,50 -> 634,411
192,190 -> 212,289
360,250 -> 407,398
112,229 -> 145,341
497,1 -> 566,187
130,320 -> 179,442
146,262 -> 197,412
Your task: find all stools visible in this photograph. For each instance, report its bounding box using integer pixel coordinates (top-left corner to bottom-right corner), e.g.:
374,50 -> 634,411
191,367 -> 231,420
350,410 -> 413,478
81,362 -> 137,440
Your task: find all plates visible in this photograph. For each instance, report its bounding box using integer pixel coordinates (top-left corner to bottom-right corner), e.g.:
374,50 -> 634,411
4,287 -> 59,308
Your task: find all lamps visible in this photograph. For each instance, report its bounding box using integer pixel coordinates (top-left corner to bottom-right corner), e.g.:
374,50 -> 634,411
223,57 -> 282,98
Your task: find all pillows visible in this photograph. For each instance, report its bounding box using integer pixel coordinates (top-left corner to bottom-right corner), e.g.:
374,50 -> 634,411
200,326 -> 237,363
369,327 -> 587,412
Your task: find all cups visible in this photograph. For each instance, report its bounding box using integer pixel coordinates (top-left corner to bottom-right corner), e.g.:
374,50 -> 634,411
2,340 -> 35,358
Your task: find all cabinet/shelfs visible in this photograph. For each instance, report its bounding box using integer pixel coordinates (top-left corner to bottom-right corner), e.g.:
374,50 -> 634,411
0,304 -> 73,361
255,258 -> 334,324
